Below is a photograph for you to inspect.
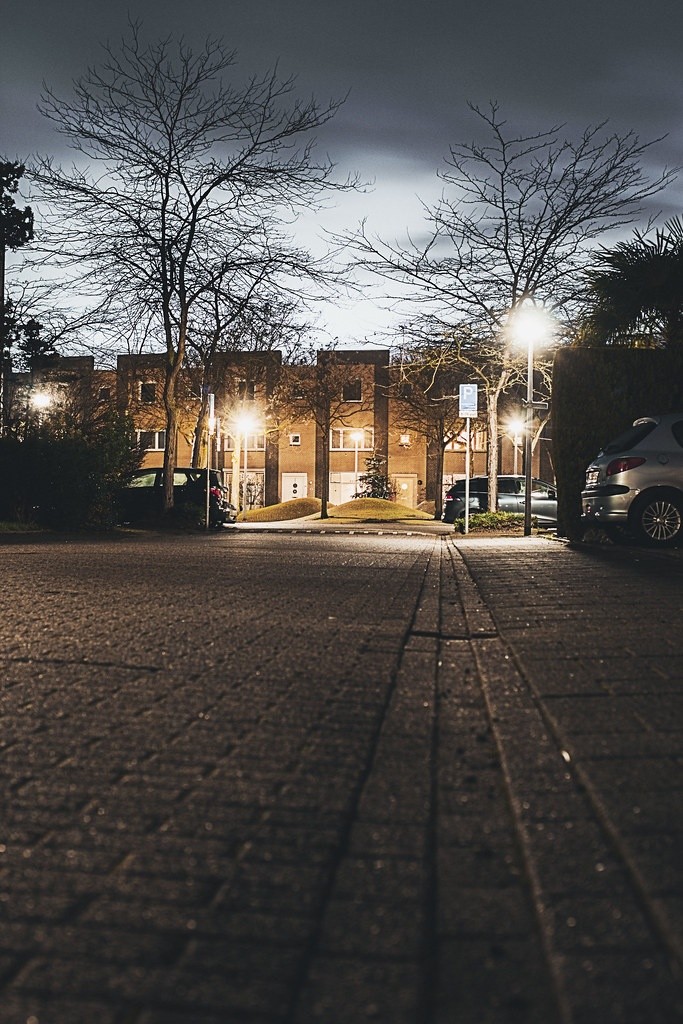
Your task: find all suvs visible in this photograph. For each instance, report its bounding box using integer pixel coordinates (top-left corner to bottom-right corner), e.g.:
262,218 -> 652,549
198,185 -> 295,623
441,476 -> 566,532
578,405 -> 683,551
102,467 -> 238,531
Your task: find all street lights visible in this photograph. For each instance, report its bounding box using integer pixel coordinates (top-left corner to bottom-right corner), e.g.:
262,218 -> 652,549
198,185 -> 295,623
34,390 -> 51,442
508,413 -> 528,474
232,410 -> 257,521
351,430 -> 363,502
515,308 -> 544,536
